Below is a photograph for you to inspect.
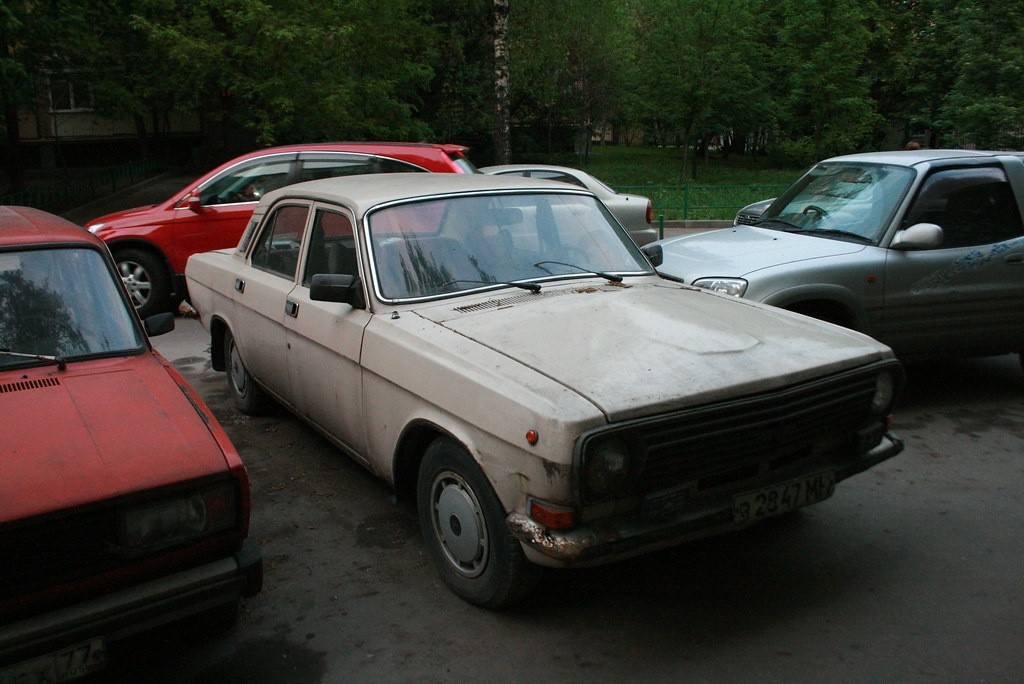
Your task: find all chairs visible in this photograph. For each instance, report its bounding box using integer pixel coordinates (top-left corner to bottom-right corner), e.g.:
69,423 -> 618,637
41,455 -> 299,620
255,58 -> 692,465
377,236 -> 483,298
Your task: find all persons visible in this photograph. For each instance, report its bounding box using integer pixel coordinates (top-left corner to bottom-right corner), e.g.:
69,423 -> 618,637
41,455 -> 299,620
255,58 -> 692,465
237,183 -> 261,202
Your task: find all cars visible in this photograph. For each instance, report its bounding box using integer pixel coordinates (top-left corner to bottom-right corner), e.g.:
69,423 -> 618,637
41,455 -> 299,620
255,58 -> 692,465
479,164 -> 661,261
0,206 -> 267,683
632,147 -> 1023,383
181,172 -> 907,614
730,165 -> 902,231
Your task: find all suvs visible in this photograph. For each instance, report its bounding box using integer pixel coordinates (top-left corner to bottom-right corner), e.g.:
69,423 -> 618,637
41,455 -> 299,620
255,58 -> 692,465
81,138 -> 512,324
